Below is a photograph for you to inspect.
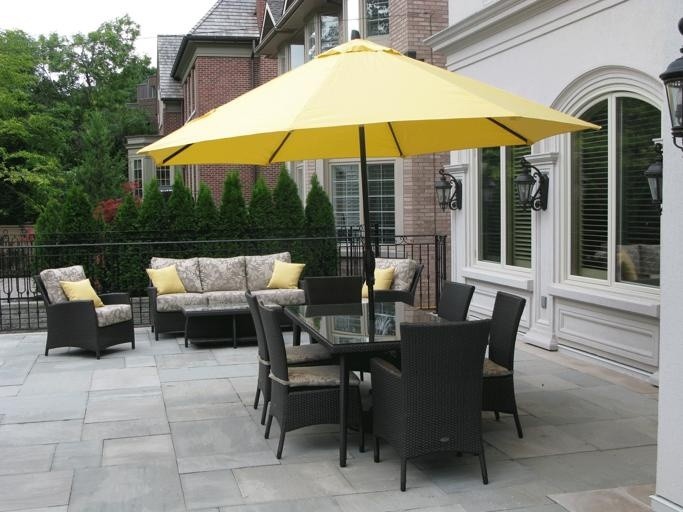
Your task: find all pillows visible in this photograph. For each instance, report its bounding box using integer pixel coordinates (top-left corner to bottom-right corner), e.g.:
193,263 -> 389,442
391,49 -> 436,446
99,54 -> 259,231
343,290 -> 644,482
40,265 -> 86,305
197,256 -> 246,292
59,279 -> 105,307
145,264 -> 189,291
151,256 -> 203,292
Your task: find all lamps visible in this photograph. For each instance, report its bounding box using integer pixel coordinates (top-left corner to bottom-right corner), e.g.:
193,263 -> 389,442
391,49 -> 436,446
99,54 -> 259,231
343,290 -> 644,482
513,157 -> 548,210
659,16 -> 683,151
435,169 -> 462,213
643,144 -> 663,217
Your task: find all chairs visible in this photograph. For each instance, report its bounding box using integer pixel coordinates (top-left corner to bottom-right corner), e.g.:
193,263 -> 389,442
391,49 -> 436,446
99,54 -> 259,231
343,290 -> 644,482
33,264 -> 134,359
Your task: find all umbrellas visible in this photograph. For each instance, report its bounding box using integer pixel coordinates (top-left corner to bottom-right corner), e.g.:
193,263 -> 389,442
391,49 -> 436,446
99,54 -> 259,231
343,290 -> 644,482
131,36 -> 605,348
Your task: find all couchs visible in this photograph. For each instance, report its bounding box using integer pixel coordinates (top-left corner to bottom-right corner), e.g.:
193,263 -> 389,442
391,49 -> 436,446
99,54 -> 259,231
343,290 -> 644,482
143,251 -> 310,341
